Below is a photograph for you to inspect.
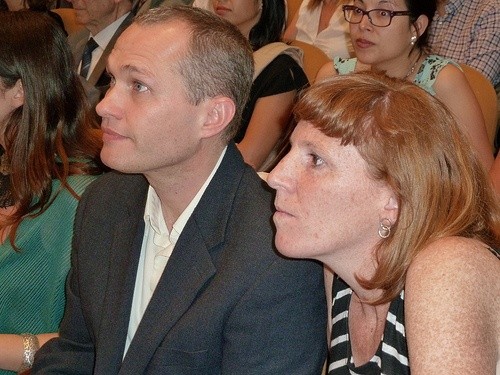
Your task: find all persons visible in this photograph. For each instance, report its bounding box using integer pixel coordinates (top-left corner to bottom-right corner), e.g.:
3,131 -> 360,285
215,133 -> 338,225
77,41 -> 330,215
0,9 -> 107,375
267,70 -> 500,374
32,3 -> 328,375
1,0 -> 499,257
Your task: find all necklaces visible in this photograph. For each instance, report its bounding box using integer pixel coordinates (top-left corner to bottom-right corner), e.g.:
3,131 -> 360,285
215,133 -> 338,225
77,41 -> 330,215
401,48 -> 423,81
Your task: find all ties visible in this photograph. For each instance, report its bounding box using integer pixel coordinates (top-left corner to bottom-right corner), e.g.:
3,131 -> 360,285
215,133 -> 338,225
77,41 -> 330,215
79,37 -> 99,78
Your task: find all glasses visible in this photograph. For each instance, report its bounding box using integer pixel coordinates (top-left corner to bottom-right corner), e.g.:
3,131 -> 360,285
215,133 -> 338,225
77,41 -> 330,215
343,5 -> 409,27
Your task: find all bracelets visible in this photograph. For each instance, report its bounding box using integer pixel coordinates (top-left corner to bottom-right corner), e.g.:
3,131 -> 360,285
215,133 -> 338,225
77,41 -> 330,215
20,332 -> 40,374
